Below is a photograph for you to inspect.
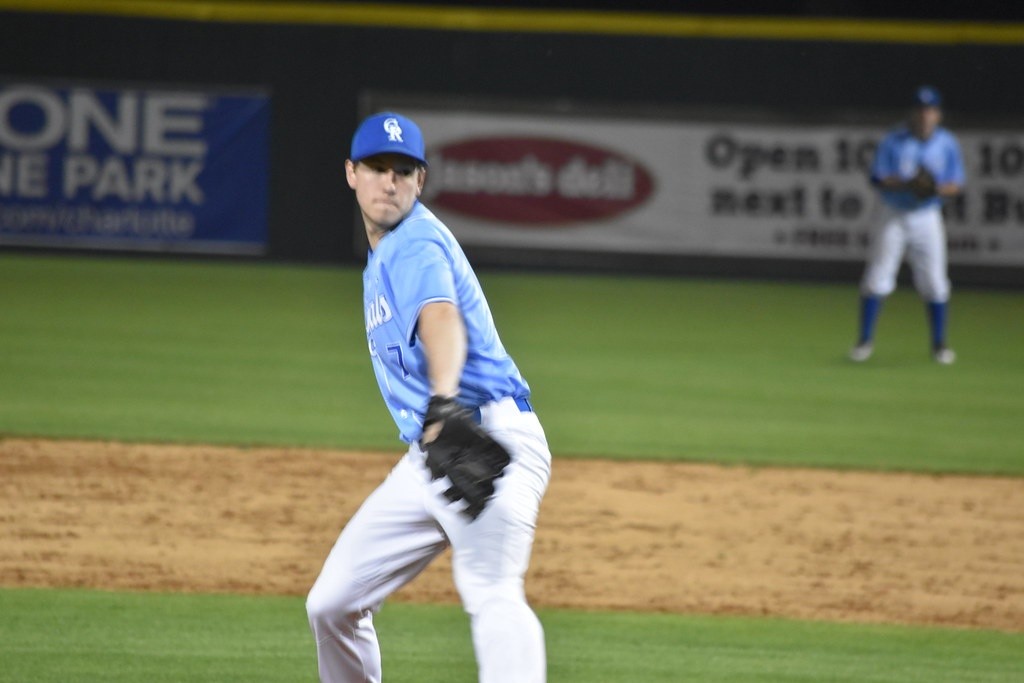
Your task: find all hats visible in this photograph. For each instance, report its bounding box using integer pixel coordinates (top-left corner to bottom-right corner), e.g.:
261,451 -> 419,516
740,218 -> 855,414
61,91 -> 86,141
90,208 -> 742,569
350,111 -> 428,168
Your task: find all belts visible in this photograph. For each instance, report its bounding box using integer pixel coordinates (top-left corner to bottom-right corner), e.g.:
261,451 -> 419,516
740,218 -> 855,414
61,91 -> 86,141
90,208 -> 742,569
472,398 -> 534,423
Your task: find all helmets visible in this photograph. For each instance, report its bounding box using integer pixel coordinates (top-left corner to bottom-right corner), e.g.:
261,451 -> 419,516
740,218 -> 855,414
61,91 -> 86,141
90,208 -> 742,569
912,88 -> 943,109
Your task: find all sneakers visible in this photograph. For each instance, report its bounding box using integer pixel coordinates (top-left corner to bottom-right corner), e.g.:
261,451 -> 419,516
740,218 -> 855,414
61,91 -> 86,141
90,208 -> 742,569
847,342 -> 871,363
932,348 -> 954,365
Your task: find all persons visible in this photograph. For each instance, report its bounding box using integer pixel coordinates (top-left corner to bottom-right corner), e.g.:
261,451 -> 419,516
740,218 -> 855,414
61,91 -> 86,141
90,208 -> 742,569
305,112 -> 552,683
848,86 -> 966,365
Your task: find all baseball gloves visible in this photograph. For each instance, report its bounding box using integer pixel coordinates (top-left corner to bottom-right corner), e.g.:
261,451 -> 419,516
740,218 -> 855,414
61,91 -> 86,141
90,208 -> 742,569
905,165 -> 938,202
416,394 -> 512,521
